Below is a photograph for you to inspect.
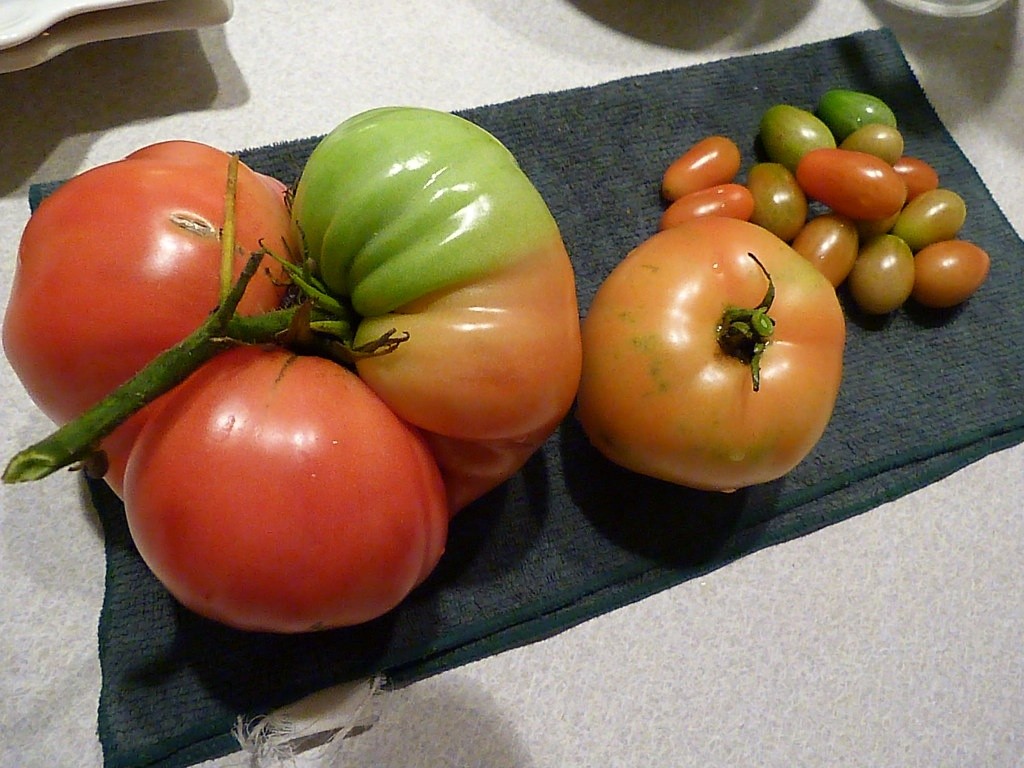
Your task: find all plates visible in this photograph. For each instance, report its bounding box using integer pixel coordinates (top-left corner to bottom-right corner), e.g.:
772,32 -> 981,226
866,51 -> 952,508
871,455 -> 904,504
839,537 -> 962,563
0,0 -> 234,74
890,0 -> 1007,18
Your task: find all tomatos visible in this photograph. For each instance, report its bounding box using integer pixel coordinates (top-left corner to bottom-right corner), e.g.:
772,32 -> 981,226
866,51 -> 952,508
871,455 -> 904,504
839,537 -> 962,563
0,105 -> 584,633
658,88 -> 991,312
573,215 -> 846,491
